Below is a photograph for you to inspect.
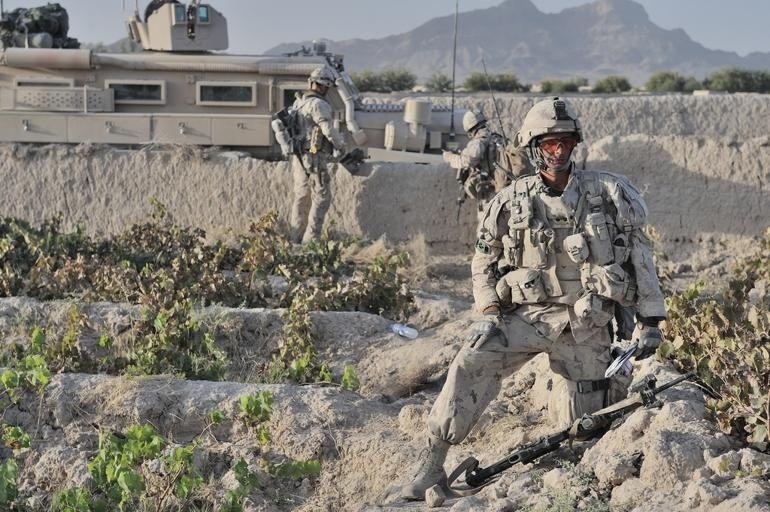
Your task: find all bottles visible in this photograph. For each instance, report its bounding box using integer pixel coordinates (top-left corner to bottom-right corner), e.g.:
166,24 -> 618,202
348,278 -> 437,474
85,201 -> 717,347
390,323 -> 418,339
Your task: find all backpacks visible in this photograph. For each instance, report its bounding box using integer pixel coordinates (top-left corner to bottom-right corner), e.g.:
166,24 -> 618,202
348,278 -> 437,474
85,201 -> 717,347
492,142 -> 538,193
270,97 -> 318,157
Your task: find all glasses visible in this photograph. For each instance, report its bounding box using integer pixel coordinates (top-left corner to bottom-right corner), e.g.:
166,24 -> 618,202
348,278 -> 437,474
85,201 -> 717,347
536,138 -> 579,152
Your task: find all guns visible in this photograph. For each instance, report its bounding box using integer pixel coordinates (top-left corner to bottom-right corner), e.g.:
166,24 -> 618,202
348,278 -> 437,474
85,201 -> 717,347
466,371 -> 694,487
456,168 -> 469,221
340,148 -> 372,167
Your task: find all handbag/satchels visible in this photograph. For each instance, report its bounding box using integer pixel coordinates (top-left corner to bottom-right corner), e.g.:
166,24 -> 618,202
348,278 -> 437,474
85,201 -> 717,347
463,171 -> 493,200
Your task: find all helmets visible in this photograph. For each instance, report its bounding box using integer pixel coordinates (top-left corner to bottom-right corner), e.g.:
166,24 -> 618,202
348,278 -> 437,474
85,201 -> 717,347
462,109 -> 488,134
513,96 -> 584,149
308,66 -> 336,88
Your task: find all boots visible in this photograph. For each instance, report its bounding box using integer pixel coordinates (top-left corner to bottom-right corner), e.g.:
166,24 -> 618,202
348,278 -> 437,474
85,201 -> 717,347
401,435 -> 451,499
611,347 -> 633,376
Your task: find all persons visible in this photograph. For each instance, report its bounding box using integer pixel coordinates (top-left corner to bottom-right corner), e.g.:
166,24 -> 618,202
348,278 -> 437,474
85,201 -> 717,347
292,66 -> 356,247
397,98 -> 670,503
455,110 -> 513,224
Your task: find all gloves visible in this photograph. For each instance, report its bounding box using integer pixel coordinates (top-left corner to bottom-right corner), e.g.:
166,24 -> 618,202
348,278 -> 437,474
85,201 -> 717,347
631,321 -> 661,361
465,311 -> 509,351
